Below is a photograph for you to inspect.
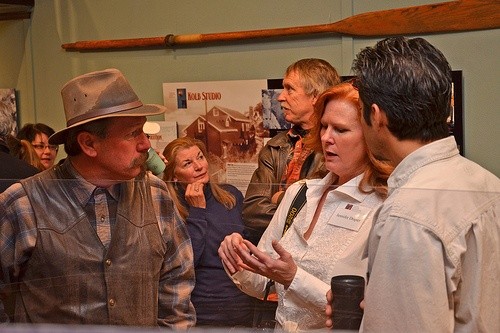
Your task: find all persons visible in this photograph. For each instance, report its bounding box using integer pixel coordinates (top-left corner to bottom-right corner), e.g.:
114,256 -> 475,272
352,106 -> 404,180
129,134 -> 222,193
0,58 -> 341,333
327,35 -> 500,333
218,78 -> 394,332
0,68 -> 197,333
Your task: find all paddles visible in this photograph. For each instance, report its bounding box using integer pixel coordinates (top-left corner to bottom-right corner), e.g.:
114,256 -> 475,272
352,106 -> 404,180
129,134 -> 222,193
62,0 -> 500,52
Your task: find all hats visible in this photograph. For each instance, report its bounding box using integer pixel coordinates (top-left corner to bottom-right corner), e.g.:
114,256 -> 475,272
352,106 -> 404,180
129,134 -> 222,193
47,68 -> 168,144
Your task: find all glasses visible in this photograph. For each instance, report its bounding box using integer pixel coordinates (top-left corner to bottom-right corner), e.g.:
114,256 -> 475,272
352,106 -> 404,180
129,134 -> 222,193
29,141 -> 60,151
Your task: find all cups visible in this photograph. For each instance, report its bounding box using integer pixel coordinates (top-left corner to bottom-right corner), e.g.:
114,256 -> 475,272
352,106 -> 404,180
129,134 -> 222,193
331,275 -> 365,329
146,148 -> 167,175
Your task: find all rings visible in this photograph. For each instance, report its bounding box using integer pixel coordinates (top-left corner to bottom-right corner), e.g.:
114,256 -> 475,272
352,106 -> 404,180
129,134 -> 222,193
194,188 -> 199,191
